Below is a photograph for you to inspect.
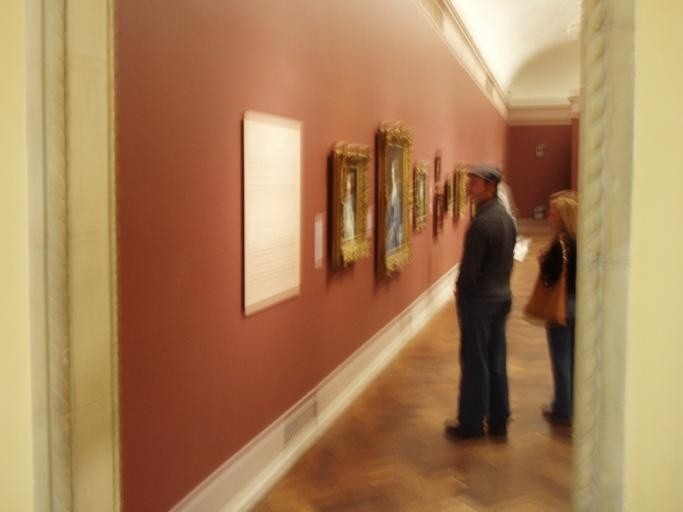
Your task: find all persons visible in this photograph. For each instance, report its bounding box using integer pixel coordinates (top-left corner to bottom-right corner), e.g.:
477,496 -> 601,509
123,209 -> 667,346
538,193 -> 578,425
445,166 -> 517,439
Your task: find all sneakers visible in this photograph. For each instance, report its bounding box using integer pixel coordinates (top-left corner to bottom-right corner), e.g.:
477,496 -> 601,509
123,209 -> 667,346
541,404 -> 572,427
445,418 -> 508,439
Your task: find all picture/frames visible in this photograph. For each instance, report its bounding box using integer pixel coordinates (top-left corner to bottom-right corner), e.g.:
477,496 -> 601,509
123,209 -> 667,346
331,128 -> 467,278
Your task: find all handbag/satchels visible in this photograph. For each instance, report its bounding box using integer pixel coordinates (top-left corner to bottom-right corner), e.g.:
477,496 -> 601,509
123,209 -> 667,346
525,281 -> 566,326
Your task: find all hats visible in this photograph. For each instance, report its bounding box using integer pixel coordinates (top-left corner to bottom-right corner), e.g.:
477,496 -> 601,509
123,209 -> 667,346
466,166 -> 502,184
